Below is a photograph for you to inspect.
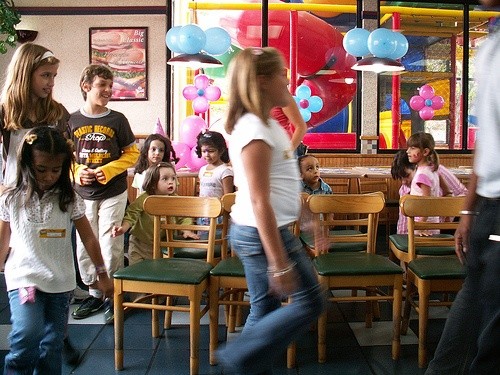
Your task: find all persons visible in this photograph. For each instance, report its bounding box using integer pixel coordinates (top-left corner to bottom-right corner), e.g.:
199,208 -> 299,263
0,43 -> 472,375
427,0 -> 500,375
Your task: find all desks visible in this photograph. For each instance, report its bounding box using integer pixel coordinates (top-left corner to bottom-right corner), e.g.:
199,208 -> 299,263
127,166 -> 473,231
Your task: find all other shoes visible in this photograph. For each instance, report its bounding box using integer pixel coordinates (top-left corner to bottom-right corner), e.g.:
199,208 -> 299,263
63,337 -> 80,366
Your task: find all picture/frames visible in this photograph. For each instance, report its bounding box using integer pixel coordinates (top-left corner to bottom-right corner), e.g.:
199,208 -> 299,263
89,26 -> 148,101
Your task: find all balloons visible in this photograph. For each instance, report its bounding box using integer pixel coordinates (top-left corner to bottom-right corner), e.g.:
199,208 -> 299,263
165,0 -> 444,172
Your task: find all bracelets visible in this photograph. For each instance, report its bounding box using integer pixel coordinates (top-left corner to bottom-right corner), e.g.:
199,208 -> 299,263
96,265 -> 108,275
459,211 -> 480,216
266,259 -> 297,276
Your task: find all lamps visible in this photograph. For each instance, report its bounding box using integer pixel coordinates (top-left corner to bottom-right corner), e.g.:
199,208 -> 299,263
167,53 -> 224,68
16,29 -> 38,44
351,56 -> 405,73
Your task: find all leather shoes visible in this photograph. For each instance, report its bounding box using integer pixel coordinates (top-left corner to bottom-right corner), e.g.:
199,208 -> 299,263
71,295 -> 103,318
105,298 -> 128,324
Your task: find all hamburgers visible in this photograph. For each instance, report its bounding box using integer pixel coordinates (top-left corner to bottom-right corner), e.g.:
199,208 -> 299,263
91,31 -> 146,91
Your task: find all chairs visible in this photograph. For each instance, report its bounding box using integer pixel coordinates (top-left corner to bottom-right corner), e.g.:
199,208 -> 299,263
113,191 -> 468,375
